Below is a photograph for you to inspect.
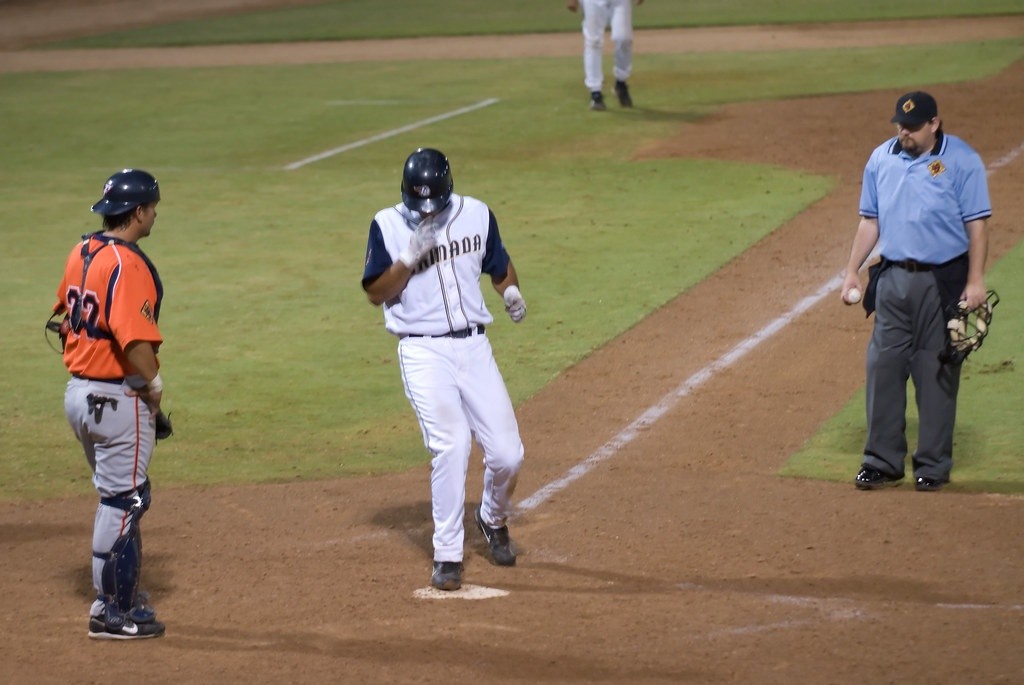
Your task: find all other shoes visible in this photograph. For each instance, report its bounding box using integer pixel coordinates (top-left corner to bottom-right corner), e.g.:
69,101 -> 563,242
915,473 -> 951,491
856,466 -> 907,489
590,95 -> 605,110
614,84 -> 631,107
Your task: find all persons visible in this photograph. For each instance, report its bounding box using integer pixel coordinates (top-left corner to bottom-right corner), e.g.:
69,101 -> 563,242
839,89 -> 991,492
566,0 -> 644,111
361,148 -> 527,591
56,168 -> 166,638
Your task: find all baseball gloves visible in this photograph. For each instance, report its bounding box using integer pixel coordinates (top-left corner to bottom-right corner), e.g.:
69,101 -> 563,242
157,409 -> 172,439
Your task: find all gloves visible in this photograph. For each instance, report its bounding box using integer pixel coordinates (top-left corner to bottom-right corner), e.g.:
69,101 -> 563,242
399,214 -> 439,269
504,285 -> 527,323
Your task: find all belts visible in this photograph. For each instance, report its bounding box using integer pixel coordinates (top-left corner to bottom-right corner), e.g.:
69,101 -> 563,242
409,326 -> 485,339
72,374 -> 125,385
890,256 -> 962,272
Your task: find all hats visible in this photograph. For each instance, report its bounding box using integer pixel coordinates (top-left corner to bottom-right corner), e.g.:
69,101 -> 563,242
890,91 -> 937,124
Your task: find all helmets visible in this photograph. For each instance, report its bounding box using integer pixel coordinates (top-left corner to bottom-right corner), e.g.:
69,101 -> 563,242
90,169 -> 159,215
400,147 -> 453,213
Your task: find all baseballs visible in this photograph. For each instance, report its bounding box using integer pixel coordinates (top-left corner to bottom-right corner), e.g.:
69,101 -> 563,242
847,288 -> 861,304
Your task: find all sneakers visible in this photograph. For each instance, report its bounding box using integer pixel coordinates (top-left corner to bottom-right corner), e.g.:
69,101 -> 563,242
127,592 -> 156,621
432,561 -> 461,591
88,615 -> 165,639
475,502 -> 516,565
